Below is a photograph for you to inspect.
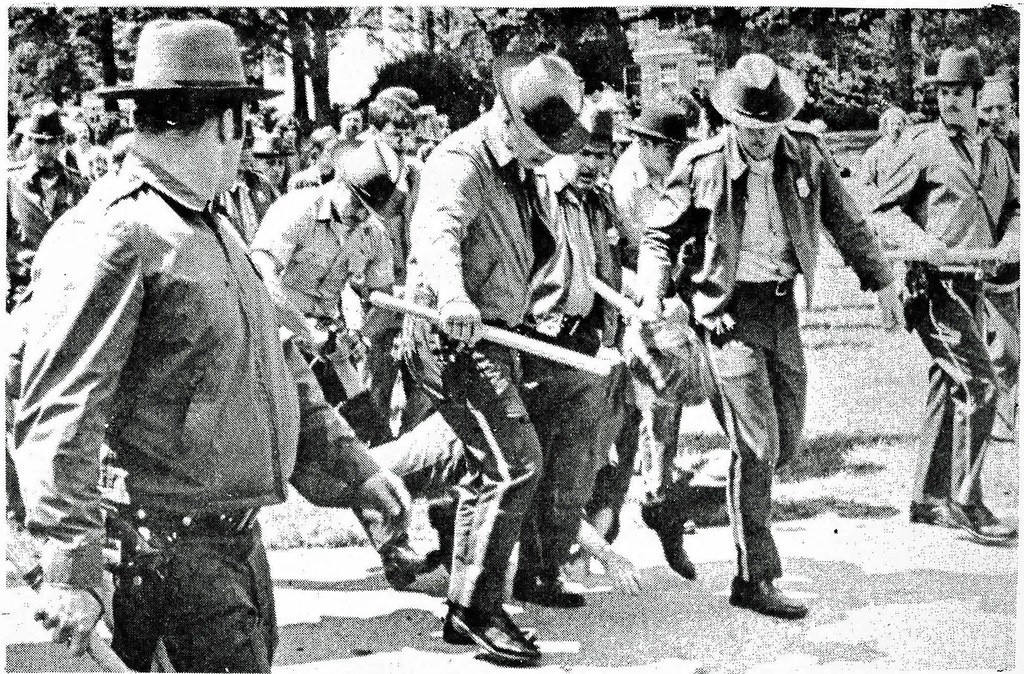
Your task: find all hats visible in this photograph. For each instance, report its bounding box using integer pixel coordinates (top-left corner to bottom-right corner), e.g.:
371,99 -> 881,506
331,136 -> 401,220
624,104 -> 696,144
491,51 -> 593,154
708,53 -> 804,128
250,134 -> 297,158
15,101 -> 72,138
928,46 -> 985,85
93,18 -> 284,99
581,106 -> 629,154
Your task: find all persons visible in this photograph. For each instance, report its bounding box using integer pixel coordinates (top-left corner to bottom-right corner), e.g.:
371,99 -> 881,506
861,105 -> 906,217
609,100 -> 700,538
973,75 -> 1019,447
872,45 -> 1019,547
7,77 -> 452,527
428,96 -> 632,610
907,111 -> 926,124
249,134 -> 443,589
658,89 -> 701,130
635,52 -> 909,620
12,18 -> 413,674
407,50 -> 588,665
264,313 -> 714,598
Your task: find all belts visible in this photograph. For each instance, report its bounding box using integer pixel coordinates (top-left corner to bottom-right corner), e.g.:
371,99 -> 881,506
103,497 -> 261,536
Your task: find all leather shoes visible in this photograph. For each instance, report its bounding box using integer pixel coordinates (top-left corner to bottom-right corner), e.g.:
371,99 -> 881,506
909,502 -> 961,528
443,615 -> 536,644
729,577 -> 807,619
944,503 -> 1016,541
512,572 -> 586,613
642,505 -> 696,580
428,503 -> 452,575
452,607 -> 540,663
382,548 -> 442,592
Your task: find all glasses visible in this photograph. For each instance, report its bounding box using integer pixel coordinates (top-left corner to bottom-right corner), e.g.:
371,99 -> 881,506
578,149 -> 606,161
